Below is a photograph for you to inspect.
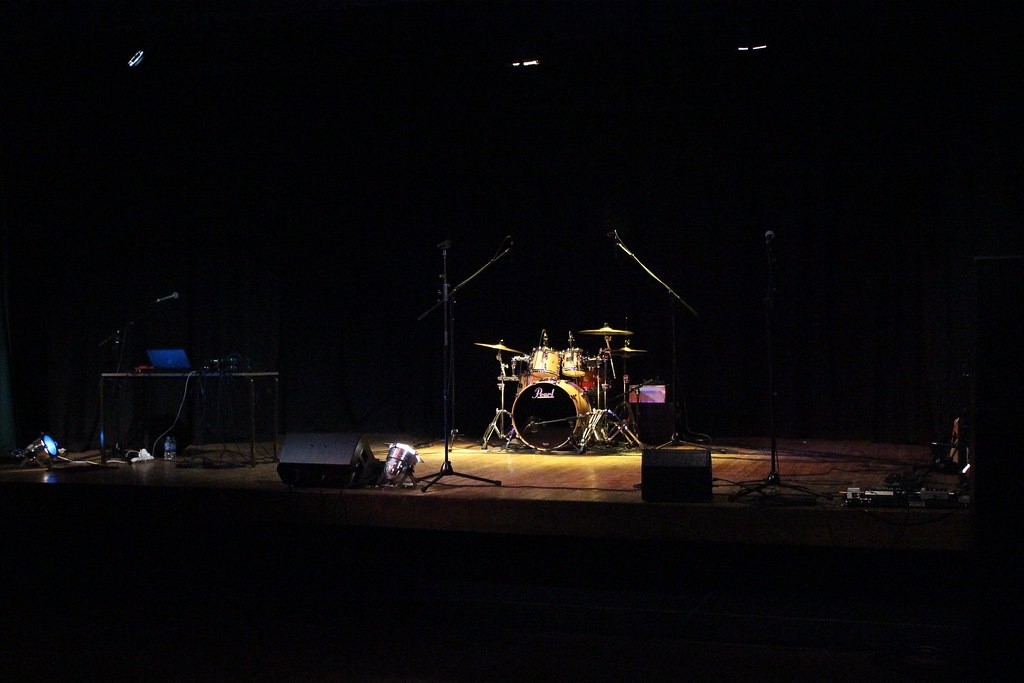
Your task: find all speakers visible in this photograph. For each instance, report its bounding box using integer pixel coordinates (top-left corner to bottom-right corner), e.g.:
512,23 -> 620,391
641,448 -> 715,504
125,410 -> 194,459
627,379 -> 685,446
278,431 -> 377,491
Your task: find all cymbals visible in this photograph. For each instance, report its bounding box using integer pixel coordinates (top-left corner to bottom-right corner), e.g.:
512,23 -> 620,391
474,342 -> 523,355
578,327 -> 633,337
611,347 -> 649,354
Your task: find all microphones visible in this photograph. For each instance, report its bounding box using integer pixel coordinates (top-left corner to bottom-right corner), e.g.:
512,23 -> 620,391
511,238 -> 513,263
569,331 -> 575,346
156,292 -> 179,302
766,231 -> 775,243
615,231 -> 618,258
544,330 -> 550,347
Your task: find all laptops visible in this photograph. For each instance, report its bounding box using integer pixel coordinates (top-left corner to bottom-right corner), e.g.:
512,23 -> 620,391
147,348 -> 199,373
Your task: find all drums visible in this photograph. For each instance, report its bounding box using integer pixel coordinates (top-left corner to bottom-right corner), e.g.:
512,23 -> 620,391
510,355 -> 531,378
511,380 -> 591,451
529,346 -> 560,378
574,358 -> 609,394
561,347 -> 588,378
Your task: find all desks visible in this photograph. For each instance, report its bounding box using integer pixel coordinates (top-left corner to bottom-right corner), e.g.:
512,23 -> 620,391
101,371 -> 279,467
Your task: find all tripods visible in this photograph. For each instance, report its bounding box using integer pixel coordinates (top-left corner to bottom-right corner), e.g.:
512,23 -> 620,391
576,242 -> 726,457
729,242 -> 835,502
410,241 -> 519,494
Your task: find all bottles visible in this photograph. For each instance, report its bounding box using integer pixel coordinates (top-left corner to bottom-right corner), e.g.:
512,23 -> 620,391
163,436 -> 176,460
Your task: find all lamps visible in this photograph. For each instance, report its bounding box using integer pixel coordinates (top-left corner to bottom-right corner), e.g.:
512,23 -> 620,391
21,434 -> 58,467
379,444 -> 419,489
